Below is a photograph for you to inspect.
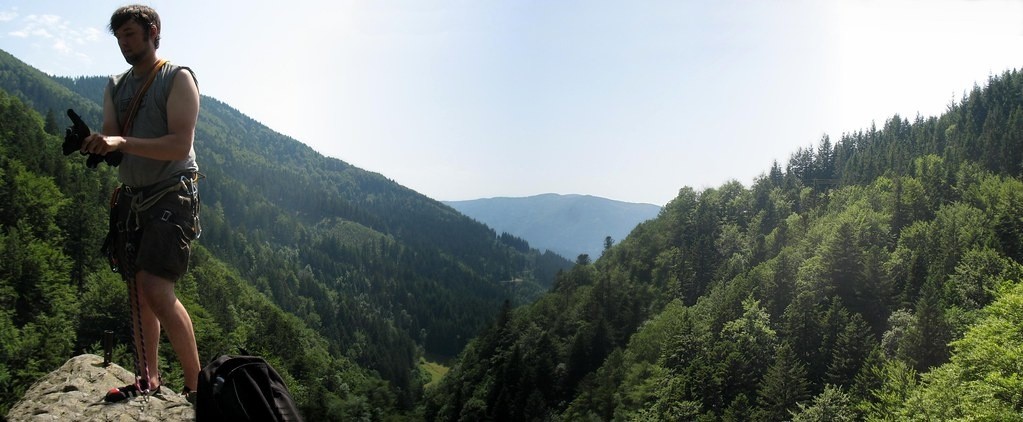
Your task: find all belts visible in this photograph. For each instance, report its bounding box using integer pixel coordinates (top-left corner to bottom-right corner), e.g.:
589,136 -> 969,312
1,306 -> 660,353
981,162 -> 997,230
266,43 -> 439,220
123,173 -> 193,195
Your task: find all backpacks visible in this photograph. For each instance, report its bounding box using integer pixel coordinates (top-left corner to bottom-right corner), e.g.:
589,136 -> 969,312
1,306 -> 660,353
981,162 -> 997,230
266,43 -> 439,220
195,353 -> 302,422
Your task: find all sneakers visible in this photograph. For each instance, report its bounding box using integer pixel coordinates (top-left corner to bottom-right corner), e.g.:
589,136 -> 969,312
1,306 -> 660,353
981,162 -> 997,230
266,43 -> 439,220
181,386 -> 197,405
105,380 -> 161,402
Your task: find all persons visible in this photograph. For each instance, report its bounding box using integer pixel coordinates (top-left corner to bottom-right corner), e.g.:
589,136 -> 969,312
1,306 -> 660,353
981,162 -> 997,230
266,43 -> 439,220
81,4 -> 202,403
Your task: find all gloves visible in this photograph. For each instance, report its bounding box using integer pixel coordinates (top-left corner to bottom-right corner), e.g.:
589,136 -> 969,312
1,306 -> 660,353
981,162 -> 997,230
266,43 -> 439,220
62,108 -> 91,157
79,142 -> 123,168
